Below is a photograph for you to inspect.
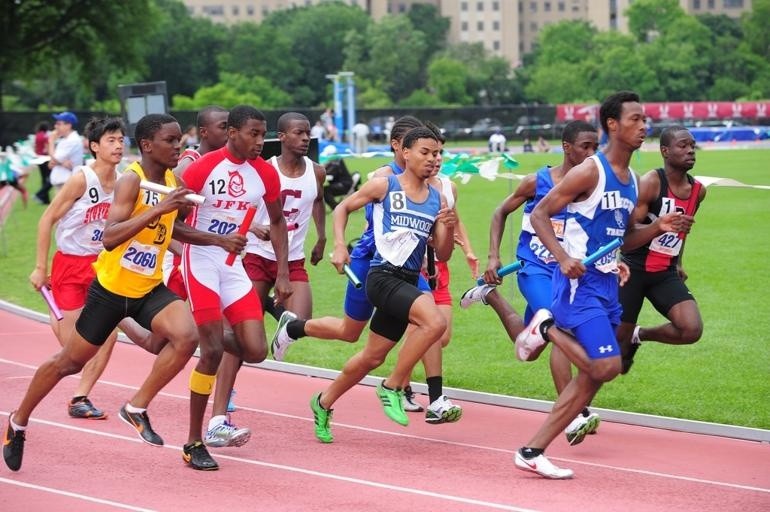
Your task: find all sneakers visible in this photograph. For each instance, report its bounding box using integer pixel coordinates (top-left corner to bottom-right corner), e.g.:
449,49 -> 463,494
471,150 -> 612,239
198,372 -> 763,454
2,410 -> 30,472
460,284 -> 496,309
621,329 -> 638,375
375,378 -> 410,426
226,388 -> 237,411
402,385 -> 424,412
515,307 -> 554,362
271,310 -> 297,361
515,448 -> 574,478
204,413 -> 252,448
310,391 -> 335,443
118,399 -> 164,447
67,396 -> 108,420
562,413 -> 600,447
182,439 -> 219,470
424,394 -> 463,424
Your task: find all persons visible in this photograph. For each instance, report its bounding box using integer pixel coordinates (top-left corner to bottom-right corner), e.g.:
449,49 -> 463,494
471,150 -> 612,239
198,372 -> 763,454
401,122 -> 481,414
269,114 -> 463,424
488,127 -> 507,153
522,138 -> 534,153
308,125 -> 458,443
459,117 -> 603,446
537,136 -> 551,152
581,125 -> 708,436
0,106 -> 372,472
511,89 -> 683,480
180,124 -> 198,148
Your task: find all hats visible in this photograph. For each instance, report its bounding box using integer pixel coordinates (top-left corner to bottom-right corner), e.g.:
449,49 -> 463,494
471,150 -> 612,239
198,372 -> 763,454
53,112 -> 78,124
